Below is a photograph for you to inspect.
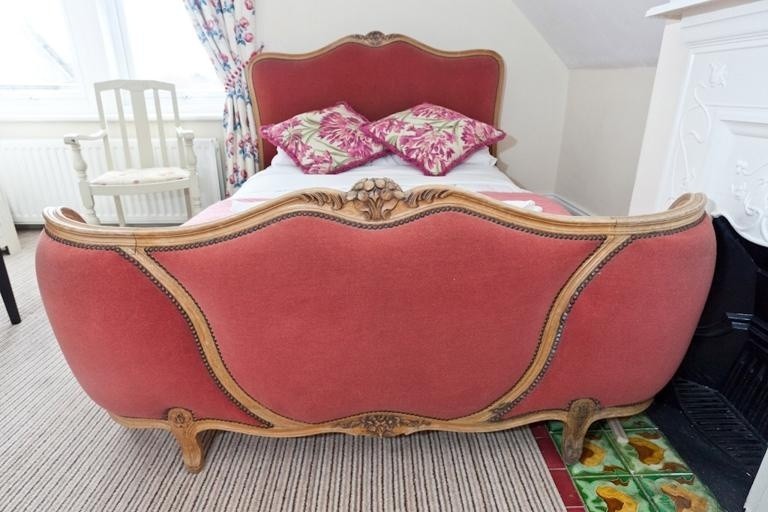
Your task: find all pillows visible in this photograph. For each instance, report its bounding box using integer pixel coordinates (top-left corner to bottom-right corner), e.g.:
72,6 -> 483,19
357,98 -> 509,177
258,101 -> 394,175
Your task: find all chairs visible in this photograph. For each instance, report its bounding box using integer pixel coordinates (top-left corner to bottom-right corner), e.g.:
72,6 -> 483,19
62,77 -> 204,229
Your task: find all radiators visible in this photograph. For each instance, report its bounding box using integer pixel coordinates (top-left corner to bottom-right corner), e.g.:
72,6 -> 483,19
1,137 -> 224,225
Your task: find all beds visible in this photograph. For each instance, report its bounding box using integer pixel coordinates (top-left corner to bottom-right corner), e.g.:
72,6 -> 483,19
27,25 -> 723,471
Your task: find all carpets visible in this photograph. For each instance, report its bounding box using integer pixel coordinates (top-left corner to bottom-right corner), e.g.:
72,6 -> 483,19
0,217 -> 571,512
